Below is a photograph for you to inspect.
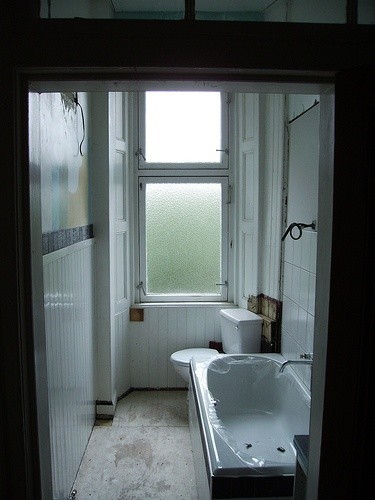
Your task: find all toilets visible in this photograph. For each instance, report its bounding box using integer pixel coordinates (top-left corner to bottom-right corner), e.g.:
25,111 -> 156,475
169,309 -> 263,405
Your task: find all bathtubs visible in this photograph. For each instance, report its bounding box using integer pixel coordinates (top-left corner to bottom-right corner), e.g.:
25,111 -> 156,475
207,355 -> 312,463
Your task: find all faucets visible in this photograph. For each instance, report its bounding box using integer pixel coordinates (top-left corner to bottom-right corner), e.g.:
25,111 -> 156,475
279,353 -> 313,391
